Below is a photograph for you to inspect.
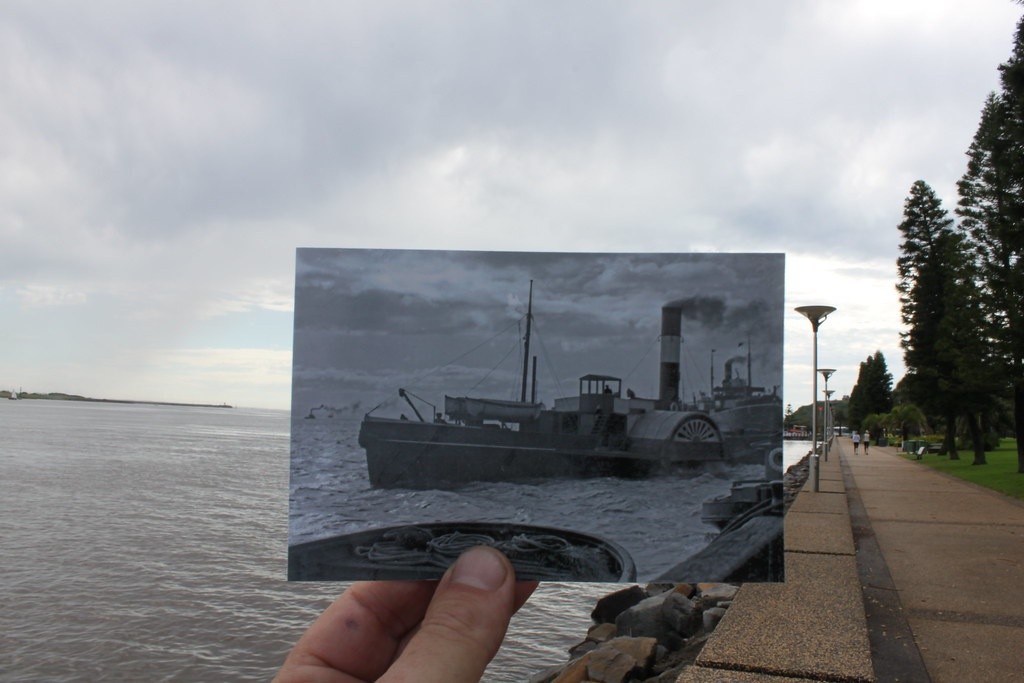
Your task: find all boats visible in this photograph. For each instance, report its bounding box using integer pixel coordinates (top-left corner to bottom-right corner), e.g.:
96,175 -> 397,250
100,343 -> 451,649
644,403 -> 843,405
289,520 -> 637,582
681,348 -> 783,466
355,278 -> 749,492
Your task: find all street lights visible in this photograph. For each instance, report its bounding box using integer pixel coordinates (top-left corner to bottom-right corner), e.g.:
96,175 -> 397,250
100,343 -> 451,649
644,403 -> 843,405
817,368 -> 837,462
822,390 -> 835,452
794,305 -> 837,493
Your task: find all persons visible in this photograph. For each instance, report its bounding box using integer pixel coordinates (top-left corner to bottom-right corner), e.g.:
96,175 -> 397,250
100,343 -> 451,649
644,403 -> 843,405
432,412 -> 446,424
603,384 -> 614,395
268,543 -> 539,682
863,430 -> 871,455
852,430 -> 861,455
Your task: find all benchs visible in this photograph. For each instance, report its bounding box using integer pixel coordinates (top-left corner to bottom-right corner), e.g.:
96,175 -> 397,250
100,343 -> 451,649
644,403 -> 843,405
913,447 -> 925,461
